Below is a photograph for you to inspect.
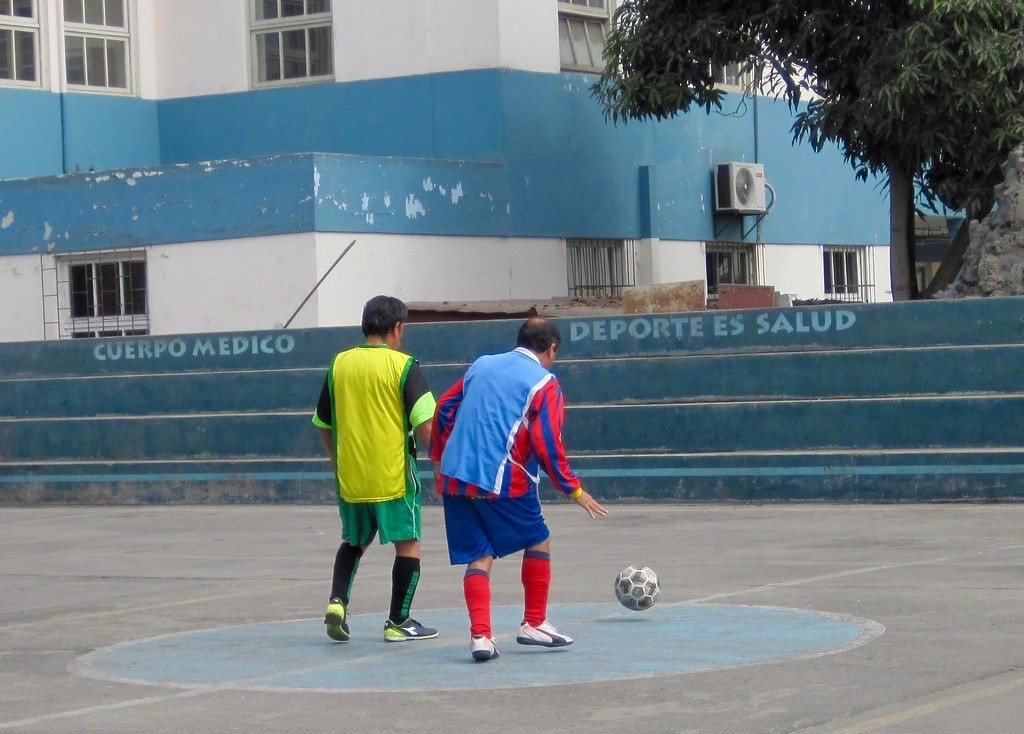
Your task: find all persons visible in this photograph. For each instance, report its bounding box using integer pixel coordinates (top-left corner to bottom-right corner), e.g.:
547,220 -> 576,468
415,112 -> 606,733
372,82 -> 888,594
429,318 -> 609,660
312,295 -> 437,642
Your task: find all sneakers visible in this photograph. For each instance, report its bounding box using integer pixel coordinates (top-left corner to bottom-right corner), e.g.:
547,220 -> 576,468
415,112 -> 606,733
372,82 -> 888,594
383,617 -> 439,641
470,635 -> 499,660
517,619 -> 574,647
324,597 -> 350,641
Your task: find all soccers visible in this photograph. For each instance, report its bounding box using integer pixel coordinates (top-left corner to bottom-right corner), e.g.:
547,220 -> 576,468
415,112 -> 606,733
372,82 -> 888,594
614,564 -> 661,611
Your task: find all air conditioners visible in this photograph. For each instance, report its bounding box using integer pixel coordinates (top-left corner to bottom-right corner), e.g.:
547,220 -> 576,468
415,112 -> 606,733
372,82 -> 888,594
713,161 -> 765,214
